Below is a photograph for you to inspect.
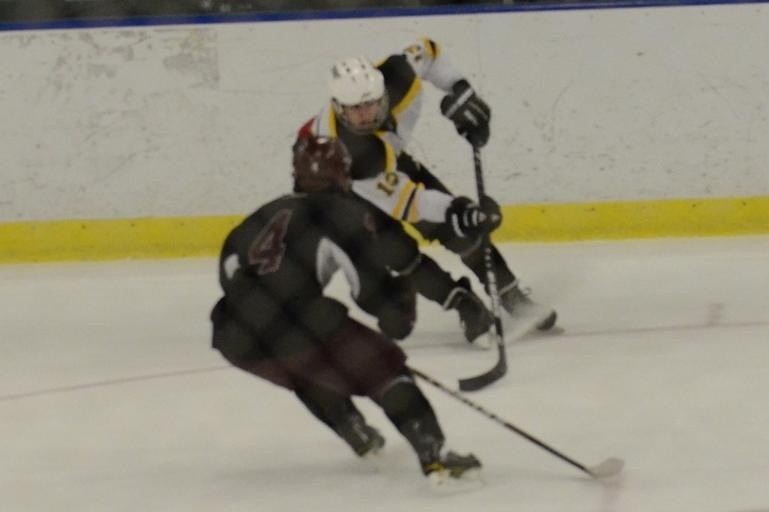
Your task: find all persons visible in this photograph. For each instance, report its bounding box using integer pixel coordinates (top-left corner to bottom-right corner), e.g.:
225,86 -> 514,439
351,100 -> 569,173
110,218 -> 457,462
292,38 -> 556,341
212,134 -> 480,478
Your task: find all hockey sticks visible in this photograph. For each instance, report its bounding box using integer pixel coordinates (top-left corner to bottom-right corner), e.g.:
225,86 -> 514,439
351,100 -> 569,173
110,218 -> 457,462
409,367 -> 625,480
458,140 -> 507,391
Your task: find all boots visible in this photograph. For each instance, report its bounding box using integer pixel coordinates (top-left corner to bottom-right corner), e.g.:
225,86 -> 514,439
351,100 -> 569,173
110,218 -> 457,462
443,279 -> 494,337
405,419 -> 481,476
497,280 -> 557,330
331,411 -> 385,455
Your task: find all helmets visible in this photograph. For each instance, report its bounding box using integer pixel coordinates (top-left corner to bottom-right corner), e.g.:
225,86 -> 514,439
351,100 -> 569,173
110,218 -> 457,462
289,134 -> 352,192
330,54 -> 389,134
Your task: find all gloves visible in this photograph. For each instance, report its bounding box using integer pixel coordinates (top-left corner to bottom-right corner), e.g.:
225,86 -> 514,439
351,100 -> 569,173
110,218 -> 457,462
438,78 -> 491,147
447,192 -> 503,237
